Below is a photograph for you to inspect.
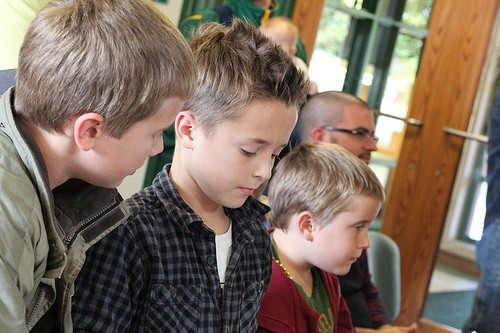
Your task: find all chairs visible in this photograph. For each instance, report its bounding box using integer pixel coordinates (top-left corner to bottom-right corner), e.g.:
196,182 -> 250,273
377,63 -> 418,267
364,231 -> 402,323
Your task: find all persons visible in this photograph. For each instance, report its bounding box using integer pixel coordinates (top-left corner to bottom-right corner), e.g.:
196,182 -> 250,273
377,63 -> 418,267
461,85 -> 500,333
72,16 -> 311,333
143,0 -> 418,333
0,0 -> 198,333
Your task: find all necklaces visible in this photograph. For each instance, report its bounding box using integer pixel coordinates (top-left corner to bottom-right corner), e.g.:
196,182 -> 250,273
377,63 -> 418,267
272,255 -> 294,281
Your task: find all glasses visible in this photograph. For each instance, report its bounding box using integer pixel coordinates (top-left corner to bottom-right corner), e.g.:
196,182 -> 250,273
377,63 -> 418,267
322,125 -> 380,143
